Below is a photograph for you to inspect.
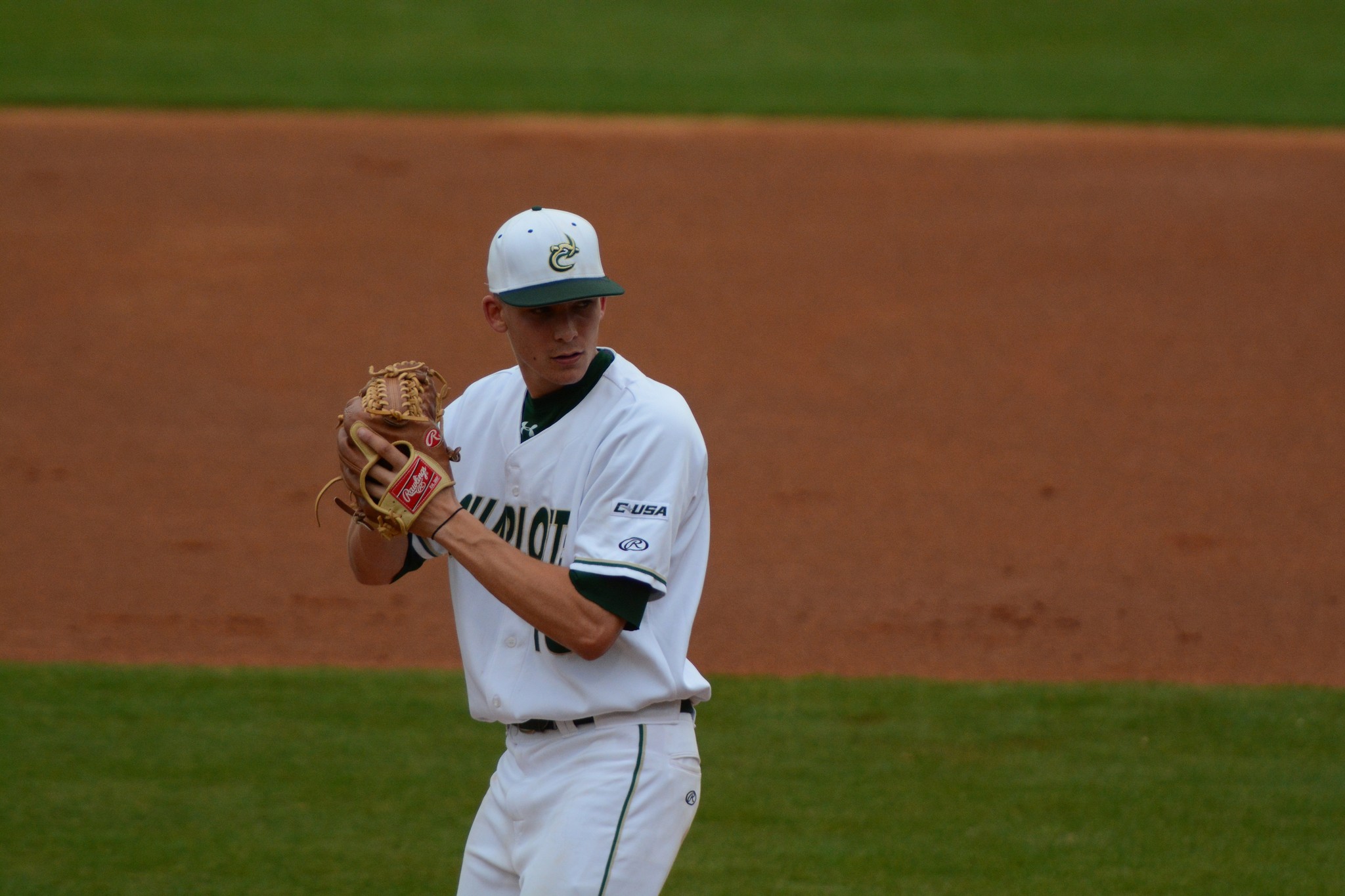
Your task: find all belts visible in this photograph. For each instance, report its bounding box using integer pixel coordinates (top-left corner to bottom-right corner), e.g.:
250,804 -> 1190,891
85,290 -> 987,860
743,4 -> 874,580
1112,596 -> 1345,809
511,697 -> 693,734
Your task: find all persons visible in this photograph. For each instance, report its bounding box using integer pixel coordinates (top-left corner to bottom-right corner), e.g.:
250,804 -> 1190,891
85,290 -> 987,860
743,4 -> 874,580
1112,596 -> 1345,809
314,207 -> 713,896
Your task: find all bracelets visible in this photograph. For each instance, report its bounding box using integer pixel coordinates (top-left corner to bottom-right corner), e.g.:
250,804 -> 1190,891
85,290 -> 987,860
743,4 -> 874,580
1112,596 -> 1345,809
430,507 -> 466,541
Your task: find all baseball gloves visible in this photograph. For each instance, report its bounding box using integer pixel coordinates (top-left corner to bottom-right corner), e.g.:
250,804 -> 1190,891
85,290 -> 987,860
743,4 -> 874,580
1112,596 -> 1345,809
339,359 -> 455,544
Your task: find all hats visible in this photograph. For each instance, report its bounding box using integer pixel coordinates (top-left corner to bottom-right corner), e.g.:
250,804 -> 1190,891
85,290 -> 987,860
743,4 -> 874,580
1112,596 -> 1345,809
486,206 -> 626,307
